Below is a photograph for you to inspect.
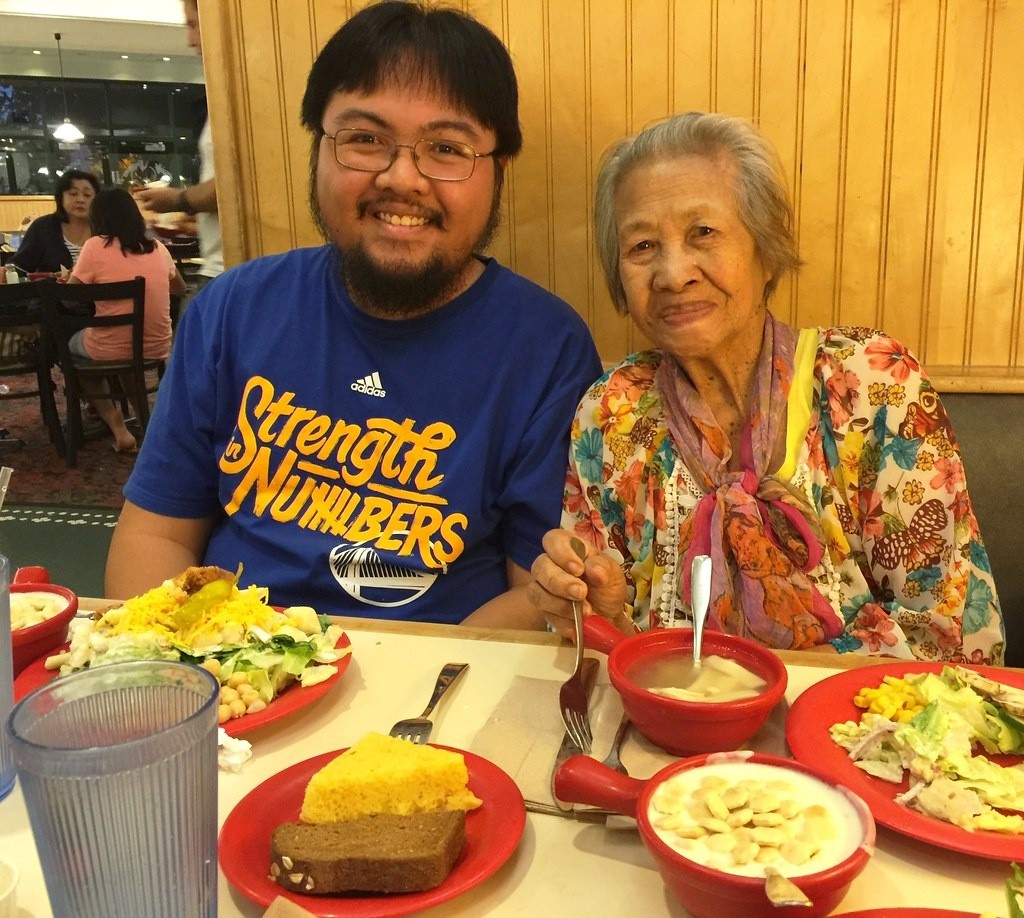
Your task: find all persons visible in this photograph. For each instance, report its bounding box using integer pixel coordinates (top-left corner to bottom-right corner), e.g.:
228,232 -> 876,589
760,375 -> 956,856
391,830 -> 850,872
135,0 -> 225,291
63,188 -> 187,453
104,0 -> 604,632
4,170 -> 101,420
526,112 -> 1006,666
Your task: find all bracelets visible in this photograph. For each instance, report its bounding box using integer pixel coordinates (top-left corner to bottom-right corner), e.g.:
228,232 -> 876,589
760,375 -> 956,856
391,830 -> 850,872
180,191 -> 197,216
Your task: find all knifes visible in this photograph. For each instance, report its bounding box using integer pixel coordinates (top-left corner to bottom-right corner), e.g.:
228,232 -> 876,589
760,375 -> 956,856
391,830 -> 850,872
550,658 -> 599,811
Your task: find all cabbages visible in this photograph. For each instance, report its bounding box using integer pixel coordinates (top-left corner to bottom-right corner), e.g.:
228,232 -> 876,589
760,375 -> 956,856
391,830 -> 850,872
831,674 -> 1023,832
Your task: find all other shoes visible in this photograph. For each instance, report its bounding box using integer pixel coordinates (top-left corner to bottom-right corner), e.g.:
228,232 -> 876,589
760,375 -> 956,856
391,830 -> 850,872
83,403 -> 98,420
113,439 -> 138,453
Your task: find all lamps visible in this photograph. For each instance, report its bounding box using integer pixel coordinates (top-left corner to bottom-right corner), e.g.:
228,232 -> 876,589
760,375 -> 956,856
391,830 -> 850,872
52,33 -> 85,143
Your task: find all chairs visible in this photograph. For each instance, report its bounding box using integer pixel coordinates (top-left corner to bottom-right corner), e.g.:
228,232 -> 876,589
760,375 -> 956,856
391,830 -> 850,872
0,242 -> 202,470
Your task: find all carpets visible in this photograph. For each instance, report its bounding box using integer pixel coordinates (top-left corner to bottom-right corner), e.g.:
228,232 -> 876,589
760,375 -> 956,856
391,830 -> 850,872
0,501 -> 197,598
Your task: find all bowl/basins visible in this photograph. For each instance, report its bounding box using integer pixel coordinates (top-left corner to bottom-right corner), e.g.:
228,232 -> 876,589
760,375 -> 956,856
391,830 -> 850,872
151,224 -> 180,237
573,614 -> 788,758
552,750 -> 877,918
26,272 -> 54,281
8,564 -> 79,680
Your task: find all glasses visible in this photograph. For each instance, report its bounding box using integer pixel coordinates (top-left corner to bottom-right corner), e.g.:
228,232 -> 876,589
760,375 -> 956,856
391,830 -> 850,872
317,123 -> 503,181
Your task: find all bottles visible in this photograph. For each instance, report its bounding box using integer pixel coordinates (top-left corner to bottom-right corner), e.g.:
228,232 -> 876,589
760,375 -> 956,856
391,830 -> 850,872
4,263 -> 20,284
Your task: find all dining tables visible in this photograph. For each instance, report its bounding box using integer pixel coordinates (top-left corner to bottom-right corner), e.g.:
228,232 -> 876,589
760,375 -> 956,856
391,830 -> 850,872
0,266 -> 199,416
0,595 -> 1024,918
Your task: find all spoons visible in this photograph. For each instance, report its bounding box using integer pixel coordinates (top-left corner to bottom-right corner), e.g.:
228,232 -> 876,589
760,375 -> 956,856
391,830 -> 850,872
601,711 -> 629,776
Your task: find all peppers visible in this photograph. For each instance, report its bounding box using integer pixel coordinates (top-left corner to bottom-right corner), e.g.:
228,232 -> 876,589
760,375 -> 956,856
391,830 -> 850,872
173,562 -> 243,627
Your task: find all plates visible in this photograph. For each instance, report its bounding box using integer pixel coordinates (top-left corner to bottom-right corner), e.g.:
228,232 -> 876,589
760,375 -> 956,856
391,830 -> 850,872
12,605 -> 352,735
784,661 -> 1024,862
218,744 -> 527,918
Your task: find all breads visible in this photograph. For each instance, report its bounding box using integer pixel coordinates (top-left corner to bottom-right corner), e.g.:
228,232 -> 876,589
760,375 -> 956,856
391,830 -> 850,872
268,808 -> 465,896
300,730 -> 482,825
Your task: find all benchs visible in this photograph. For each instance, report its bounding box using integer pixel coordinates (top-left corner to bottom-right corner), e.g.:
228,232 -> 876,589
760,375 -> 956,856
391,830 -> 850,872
924,393 -> 1024,670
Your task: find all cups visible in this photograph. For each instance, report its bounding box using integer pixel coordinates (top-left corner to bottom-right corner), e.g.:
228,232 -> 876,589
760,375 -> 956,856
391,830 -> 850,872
0,554 -> 23,802
0,267 -> 7,284
144,181 -> 169,188
8,660 -> 217,917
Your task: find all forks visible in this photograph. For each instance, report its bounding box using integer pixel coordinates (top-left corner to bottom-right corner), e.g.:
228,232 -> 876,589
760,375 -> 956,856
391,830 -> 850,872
558,537 -> 593,754
387,662 -> 469,746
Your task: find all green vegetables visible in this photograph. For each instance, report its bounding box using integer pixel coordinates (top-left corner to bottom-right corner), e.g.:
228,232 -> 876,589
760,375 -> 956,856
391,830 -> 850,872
53,614 -> 354,701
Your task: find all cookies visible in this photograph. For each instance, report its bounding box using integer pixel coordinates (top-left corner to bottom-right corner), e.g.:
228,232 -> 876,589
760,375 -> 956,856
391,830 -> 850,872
652,773 -> 833,868
